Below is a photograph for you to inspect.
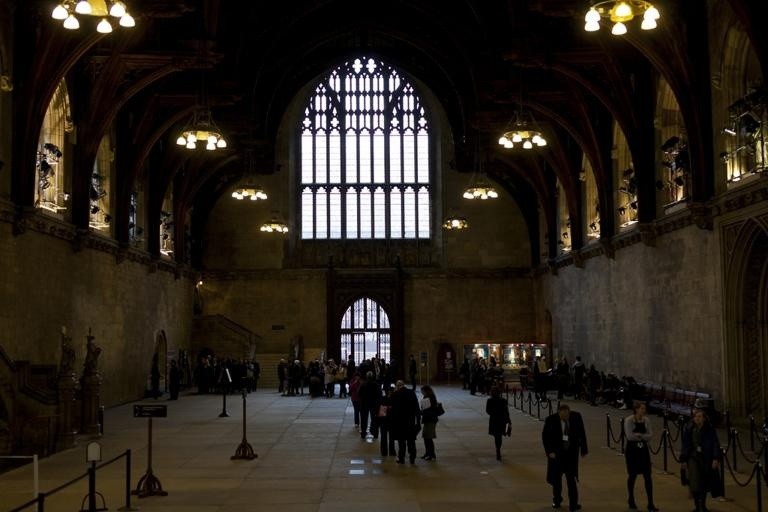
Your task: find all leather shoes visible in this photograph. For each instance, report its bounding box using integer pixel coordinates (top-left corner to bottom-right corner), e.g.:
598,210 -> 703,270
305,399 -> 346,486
381,451 -> 416,465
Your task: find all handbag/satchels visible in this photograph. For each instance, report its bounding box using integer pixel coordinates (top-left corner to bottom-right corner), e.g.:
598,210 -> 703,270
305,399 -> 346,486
436,402 -> 445,416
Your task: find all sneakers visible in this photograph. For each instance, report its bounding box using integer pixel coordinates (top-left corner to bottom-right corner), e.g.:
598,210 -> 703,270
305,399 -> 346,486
627,498 -> 637,509
568,503 -> 581,512
552,495 -> 563,508
646,502 -> 659,512
355,423 -> 378,439
495,452 -> 502,461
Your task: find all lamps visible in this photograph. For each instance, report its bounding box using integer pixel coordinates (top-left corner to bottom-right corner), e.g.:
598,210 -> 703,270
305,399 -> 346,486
171,99 -> 290,236
579,0 -> 665,37
51,0 -> 137,37
438,101 -> 549,230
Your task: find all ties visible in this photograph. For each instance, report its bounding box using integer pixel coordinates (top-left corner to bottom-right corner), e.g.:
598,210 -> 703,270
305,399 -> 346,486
564,420 -> 569,436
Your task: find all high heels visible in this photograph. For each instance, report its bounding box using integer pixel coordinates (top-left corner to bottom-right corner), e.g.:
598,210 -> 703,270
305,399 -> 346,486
420,453 -> 437,461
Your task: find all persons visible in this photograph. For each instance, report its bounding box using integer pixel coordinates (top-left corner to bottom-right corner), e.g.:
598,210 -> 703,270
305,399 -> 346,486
152,348 -> 419,407
353,386 -> 440,466
623,400 -> 660,511
540,403 -> 589,511
677,407 -> 724,512
484,386 -> 512,461
444,352 -> 639,412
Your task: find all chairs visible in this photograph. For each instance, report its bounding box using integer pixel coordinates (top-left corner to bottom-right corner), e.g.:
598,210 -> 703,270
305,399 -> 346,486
632,382 -> 710,424
